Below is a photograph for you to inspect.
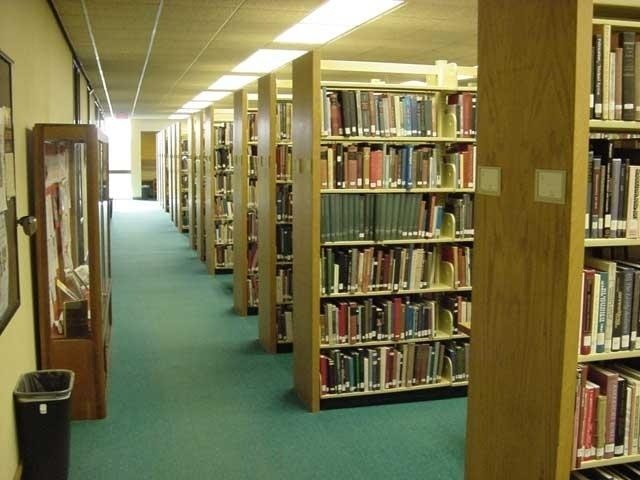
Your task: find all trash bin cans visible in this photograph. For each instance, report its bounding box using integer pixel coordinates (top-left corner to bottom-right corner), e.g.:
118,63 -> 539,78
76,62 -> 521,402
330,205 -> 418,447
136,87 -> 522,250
13,370 -> 75,479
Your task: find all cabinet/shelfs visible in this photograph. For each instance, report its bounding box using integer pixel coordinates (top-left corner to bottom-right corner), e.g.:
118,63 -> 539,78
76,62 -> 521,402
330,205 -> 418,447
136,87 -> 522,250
245,107 -> 260,313
32,117 -> 113,424
178,132 -> 189,233
275,98 -> 295,350
155,123 -> 177,230
195,112 -> 205,260
188,117 -> 196,252
321,82 -> 482,397
211,120 -> 233,273
570,0 -> 640,480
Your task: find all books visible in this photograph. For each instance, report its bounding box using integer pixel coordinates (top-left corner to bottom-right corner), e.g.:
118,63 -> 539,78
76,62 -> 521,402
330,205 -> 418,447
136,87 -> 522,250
320,89 -> 478,138
319,192 -> 476,243
181,139 -> 189,227
275,101 -> 293,143
247,241 -> 258,270
319,140 -> 477,191
571,462 -> 639,480
276,184 -> 293,223
246,212 -> 258,242
214,121 -> 234,269
275,305 -> 294,343
571,361 -> 640,468
578,246 -> 640,357
247,178 -> 258,211
246,270 -> 260,308
275,265 -> 294,303
247,143 -> 259,177
319,290 -> 473,346
56,264 -> 90,338
589,23 -> 640,124
247,113 -> 259,142
319,338 -> 471,396
584,140 -> 640,242
275,144 -> 293,182
275,223 -> 293,263
318,243 -> 473,297
